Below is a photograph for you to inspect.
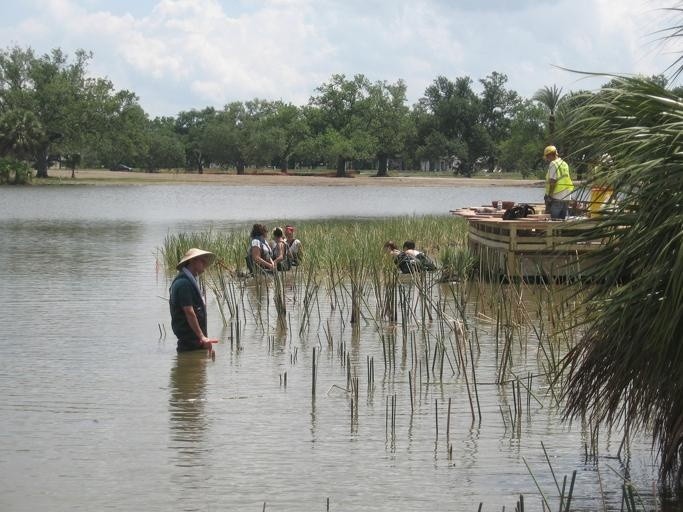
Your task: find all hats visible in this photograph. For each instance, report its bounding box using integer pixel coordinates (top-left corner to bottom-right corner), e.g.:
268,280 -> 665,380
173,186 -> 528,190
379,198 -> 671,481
176,248 -> 214,268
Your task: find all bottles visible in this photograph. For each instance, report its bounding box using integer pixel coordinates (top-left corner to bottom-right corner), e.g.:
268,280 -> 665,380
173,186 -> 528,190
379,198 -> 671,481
498,199 -> 502,212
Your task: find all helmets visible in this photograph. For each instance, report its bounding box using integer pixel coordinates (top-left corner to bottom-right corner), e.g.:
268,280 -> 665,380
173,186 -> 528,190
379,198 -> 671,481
544,146 -> 556,157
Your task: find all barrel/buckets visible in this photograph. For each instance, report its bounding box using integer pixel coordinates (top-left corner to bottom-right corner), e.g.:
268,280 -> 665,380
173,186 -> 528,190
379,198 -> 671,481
590,186 -> 613,219
547,199 -> 569,221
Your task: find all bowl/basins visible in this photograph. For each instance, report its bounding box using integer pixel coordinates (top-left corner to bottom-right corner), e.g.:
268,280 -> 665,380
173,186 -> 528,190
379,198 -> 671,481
491,201 -> 514,210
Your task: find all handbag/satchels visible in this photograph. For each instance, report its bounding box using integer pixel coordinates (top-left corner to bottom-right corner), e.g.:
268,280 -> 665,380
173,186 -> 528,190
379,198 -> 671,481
503,203 -> 534,219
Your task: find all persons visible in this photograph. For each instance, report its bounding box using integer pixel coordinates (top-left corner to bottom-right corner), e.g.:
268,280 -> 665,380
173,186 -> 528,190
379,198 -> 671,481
383,240 -> 402,271
248,223 -> 303,275
394,239 -> 437,274
168,248 -> 217,355
543,146 -> 574,219
590,154 -> 617,212
